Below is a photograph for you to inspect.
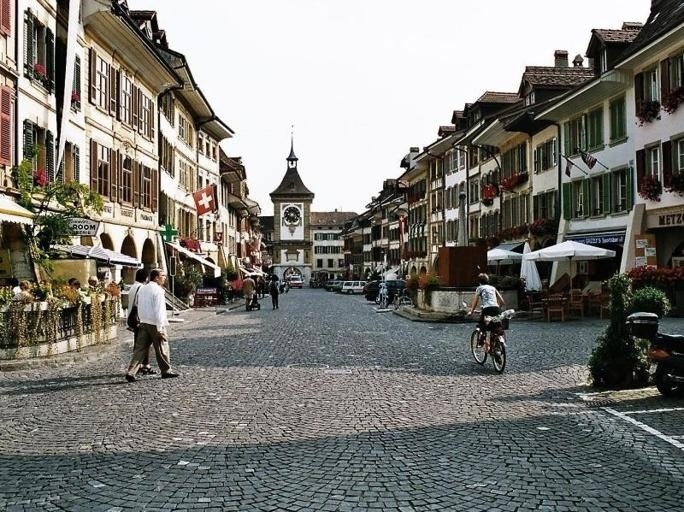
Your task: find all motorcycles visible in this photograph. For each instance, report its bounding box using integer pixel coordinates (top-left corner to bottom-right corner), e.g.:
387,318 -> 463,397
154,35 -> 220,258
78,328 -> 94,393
625,311 -> 684,398
375,284 -> 389,310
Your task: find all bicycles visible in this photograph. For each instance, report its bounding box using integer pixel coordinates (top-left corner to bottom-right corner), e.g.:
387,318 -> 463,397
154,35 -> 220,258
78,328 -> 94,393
393,288 -> 414,311
462,302 -> 507,373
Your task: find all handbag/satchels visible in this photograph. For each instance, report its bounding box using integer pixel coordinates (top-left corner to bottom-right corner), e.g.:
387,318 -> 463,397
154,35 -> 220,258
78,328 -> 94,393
128,306 -> 139,328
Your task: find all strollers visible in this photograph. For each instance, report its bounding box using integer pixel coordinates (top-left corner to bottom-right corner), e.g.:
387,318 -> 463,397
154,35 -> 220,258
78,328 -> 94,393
246,290 -> 260,311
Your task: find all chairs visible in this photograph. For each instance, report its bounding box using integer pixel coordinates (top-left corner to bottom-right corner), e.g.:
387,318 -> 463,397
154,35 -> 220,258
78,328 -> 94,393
526,282 -> 610,322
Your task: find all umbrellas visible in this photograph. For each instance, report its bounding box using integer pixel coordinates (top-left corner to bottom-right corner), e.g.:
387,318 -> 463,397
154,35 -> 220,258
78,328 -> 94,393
524,239 -> 617,303
520,240 -> 543,293
486,248 -> 525,276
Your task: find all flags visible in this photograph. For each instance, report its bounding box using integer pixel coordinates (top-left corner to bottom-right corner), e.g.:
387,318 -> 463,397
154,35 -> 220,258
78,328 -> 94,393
398,215 -> 405,223
566,160 -> 574,177
580,150 -> 598,170
191,185 -> 217,217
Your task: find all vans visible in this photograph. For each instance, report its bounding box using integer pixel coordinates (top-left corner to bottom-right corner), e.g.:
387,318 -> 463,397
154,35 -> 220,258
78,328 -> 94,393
326,280 -> 406,300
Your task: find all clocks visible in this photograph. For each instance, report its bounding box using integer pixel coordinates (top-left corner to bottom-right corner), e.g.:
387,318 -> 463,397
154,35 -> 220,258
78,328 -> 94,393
282,204 -> 301,226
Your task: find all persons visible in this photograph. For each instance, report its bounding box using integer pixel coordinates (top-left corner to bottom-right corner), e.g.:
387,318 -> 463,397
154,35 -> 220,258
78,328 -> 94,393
242,276 -> 258,309
257,277 -> 266,299
13,275 -> 98,301
124,267 -> 183,383
269,275 -> 280,310
127,270 -> 156,374
467,273 -> 506,364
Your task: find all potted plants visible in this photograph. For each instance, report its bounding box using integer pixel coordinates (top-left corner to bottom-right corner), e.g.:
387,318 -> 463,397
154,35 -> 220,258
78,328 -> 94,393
174,275 -> 197,306
635,99 -> 659,128
665,172 -> 683,196
661,83 -> 684,114
639,174 -> 662,201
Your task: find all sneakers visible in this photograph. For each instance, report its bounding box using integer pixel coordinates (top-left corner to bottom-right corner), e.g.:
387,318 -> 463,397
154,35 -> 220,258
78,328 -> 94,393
163,374 -> 177,378
126,375 -> 135,382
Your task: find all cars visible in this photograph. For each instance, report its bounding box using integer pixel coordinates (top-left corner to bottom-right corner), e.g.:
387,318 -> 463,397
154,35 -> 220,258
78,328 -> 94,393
288,276 -> 304,288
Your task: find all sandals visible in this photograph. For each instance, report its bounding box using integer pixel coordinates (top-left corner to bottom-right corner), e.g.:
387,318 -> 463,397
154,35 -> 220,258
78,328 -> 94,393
138,368 -> 156,374
